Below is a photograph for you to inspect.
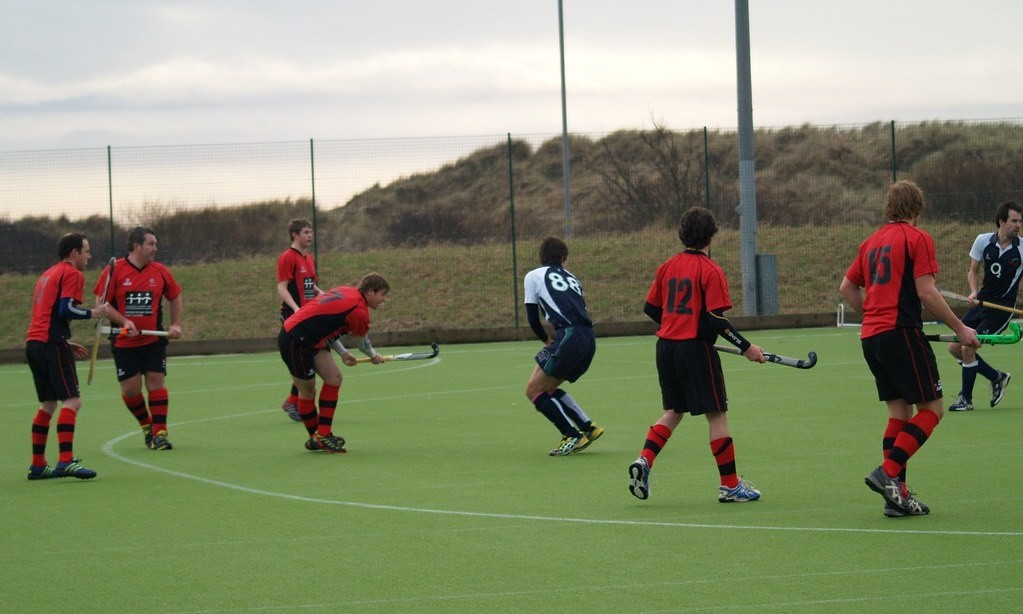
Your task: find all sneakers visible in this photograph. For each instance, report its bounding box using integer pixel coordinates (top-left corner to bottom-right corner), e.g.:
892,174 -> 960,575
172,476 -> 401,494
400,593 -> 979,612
311,431 -> 346,454
27,461 -> 64,480
304,432 -> 345,450
282,399 -> 303,421
572,422 -> 604,453
989,370 -> 1012,407
151,430 -> 172,451
55,458 -> 97,480
883,490 -> 931,518
948,392 -> 974,411
718,481 -> 761,503
549,428 -> 589,456
864,466 -> 922,514
628,457 -> 650,500
140,424 -> 152,448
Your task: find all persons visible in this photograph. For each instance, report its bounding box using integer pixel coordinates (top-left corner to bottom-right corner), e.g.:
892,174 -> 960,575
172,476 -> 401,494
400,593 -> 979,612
93,226 -> 183,451
25,232 -> 112,480
524,237 -> 604,457
277,272 -> 391,453
947,201 -> 1023,411
838,180 -> 980,517
275,219 -> 323,422
628,209 -> 767,504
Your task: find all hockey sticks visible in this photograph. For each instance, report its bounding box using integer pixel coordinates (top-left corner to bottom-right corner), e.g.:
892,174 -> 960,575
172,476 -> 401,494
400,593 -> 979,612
927,323 -> 1022,343
344,343 -> 439,366
714,345 -> 818,369
94,323 -> 171,335
936,285 -> 1022,317
87,257 -> 116,386
